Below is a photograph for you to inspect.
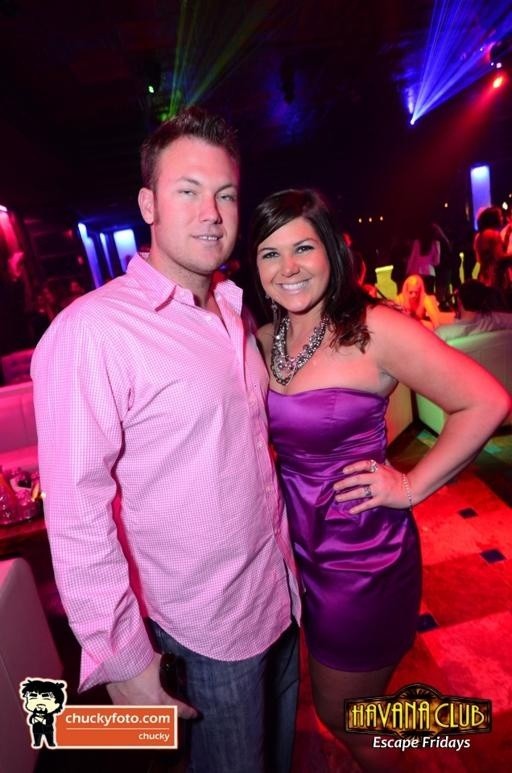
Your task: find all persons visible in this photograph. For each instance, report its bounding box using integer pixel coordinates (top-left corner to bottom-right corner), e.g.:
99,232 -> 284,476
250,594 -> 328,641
245,185 -> 512,769
0,202 -> 277,351
328,199 -> 511,334
28,105 -> 416,770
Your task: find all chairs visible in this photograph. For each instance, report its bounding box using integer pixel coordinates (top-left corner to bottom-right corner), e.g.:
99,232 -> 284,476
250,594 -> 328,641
416,326 -> 512,439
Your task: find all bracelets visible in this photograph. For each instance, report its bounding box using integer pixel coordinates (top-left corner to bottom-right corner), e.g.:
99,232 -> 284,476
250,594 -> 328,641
401,471 -> 413,514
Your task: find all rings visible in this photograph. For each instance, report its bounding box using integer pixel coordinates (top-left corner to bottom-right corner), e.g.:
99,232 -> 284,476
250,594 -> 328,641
367,458 -> 379,474
362,485 -> 372,498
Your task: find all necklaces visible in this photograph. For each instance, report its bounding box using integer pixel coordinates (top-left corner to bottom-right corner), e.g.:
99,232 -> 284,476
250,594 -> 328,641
265,309 -> 331,387
